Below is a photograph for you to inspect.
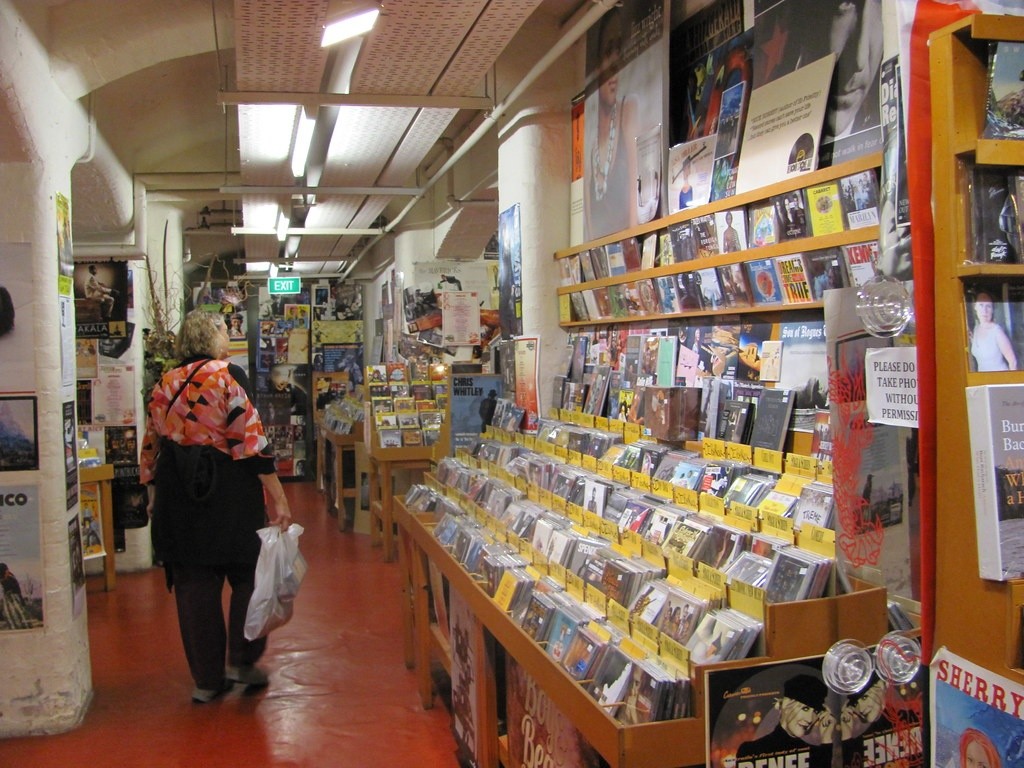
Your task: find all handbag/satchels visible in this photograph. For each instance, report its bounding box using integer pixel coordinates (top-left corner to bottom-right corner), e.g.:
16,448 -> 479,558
244,523 -> 304,641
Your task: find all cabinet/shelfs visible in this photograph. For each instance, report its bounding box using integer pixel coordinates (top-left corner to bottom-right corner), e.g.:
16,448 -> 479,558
362,364 -> 432,561
551,149 -> 880,329
314,384 -> 364,531
430,375 -> 503,465
928,13 -> 1024,768
393,416 -> 887,767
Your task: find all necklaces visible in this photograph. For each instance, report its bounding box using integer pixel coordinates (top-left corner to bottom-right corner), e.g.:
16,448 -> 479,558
593,99 -> 618,201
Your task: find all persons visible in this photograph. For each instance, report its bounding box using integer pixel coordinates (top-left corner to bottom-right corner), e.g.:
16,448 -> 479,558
969,288 -> 1019,372
193,282 -> 247,337
840,667 -> 925,767
138,306 -> 292,703
957,727 -> 1001,768
736,673 -> 838,768
84,265 -> 118,320
585,7 -> 643,241
798,0 -> 884,146
257,156 -> 910,727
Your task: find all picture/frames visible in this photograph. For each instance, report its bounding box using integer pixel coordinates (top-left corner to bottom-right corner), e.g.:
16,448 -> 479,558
0,397 -> 41,473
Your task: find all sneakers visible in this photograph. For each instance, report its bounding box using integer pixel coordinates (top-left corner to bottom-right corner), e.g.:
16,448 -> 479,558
225,661 -> 272,685
190,677 -> 235,703
